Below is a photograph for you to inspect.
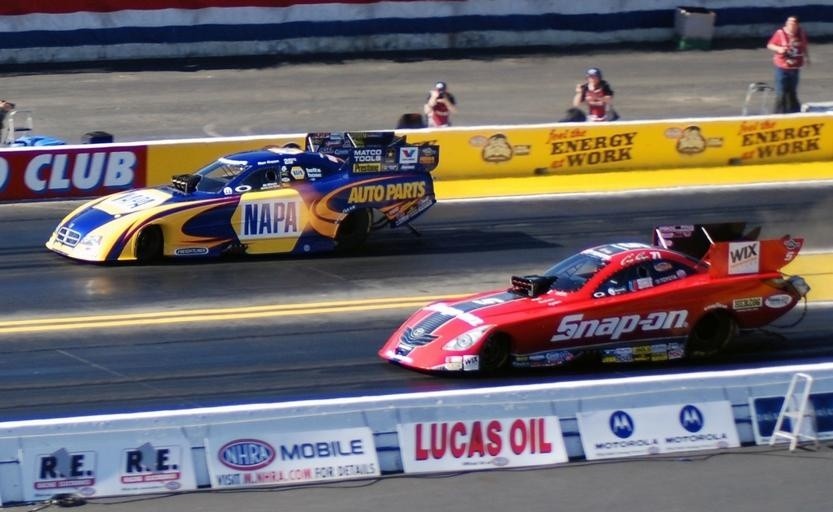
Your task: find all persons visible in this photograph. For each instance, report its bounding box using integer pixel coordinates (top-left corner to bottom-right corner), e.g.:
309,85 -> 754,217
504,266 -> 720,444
573,67 -> 616,122
0,100 -> 17,131
421,82 -> 459,128
765,16 -> 811,115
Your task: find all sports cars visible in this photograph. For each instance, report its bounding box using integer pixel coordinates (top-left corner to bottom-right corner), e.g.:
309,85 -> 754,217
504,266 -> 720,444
375,220 -> 811,379
42,131 -> 440,267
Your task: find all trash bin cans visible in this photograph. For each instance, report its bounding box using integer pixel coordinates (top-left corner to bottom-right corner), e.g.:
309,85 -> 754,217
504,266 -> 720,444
675,7 -> 716,51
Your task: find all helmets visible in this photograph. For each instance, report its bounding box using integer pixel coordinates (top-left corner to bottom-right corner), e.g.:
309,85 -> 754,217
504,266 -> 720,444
584,68 -> 602,79
434,82 -> 446,92
